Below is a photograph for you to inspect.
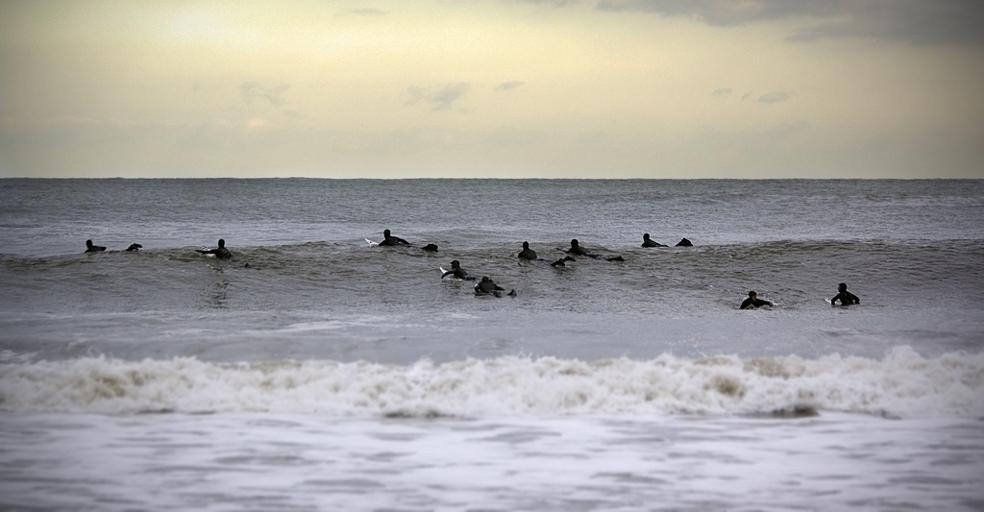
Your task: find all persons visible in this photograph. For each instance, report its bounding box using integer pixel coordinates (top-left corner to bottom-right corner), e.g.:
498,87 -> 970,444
82,239 -> 142,253
440,259 -> 476,281
517,241 -> 576,267
193,238 -> 231,260
377,229 -> 438,252
739,290 -> 773,310
639,233 -> 693,247
830,283 -> 860,306
554,238 -> 624,262
473,276 -> 517,298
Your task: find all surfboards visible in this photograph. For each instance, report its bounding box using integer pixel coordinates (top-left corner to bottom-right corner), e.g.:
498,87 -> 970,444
440,267 -> 462,280
825,297 -> 842,306
203,245 -> 218,258
365,238 -> 379,247
